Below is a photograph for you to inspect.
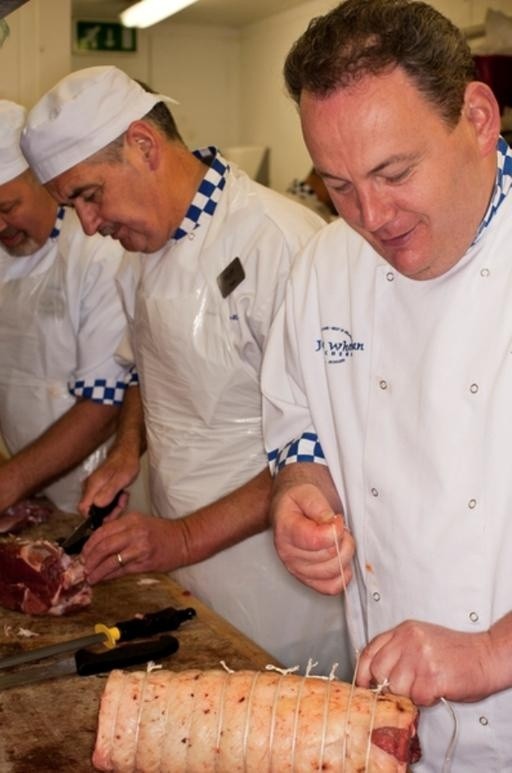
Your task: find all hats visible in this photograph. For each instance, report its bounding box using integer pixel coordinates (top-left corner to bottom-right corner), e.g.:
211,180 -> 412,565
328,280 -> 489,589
19,64 -> 158,188
0,97 -> 31,186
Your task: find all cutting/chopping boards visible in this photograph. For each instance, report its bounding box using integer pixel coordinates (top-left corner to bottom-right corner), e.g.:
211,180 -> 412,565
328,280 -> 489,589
3,495 -> 290,772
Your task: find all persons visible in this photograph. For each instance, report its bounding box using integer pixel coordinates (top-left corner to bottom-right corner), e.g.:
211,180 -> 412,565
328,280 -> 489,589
259,1 -> 512,773
1,94 -> 131,519
17,63 -> 363,685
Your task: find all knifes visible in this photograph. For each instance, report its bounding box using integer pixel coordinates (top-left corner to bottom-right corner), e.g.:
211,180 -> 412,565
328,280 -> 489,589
62,488 -> 125,555
0,635 -> 180,687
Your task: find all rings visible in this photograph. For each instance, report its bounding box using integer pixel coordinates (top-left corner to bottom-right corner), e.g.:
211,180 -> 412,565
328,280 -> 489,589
116,552 -> 125,568
